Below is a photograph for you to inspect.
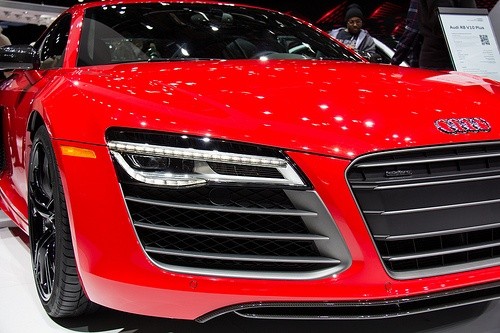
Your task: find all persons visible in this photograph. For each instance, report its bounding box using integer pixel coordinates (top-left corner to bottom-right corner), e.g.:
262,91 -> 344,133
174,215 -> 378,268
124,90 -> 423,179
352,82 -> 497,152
392,0 -> 425,69
328,3 -> 382,64
0,25 -> 15,79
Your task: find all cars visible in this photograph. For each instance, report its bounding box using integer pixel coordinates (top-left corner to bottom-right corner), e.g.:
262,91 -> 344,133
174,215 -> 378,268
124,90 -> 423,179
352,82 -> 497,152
0,0 -> 500,333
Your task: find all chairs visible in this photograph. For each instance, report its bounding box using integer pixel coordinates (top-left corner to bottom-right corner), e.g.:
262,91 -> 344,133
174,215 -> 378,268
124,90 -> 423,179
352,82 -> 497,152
221,38 -> 258,58
102,39 -> 157,62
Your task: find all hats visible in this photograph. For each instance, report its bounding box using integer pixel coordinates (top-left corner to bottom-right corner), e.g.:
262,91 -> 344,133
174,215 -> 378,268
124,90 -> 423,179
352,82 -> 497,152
345,4 -> 364,22
